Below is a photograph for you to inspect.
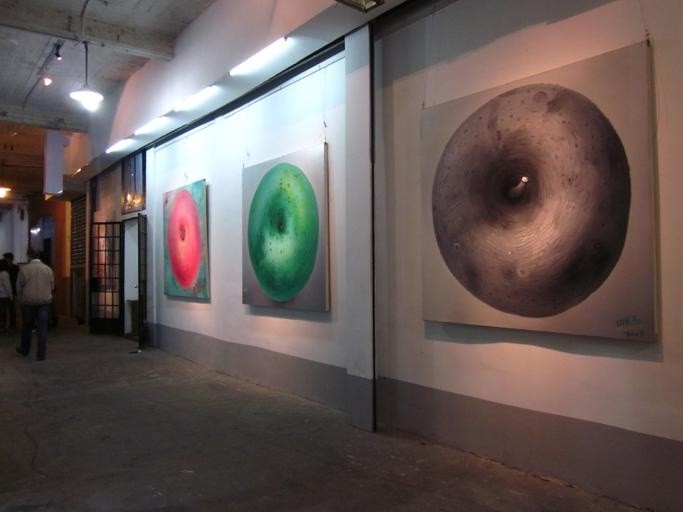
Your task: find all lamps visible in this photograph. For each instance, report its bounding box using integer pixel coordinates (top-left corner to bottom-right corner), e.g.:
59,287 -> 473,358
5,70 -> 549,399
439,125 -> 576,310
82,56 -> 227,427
34,42 -> 63,86
67,44 -> 104,110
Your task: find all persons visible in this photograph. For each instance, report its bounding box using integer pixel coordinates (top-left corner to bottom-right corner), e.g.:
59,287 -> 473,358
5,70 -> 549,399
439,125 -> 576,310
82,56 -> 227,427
0,252 -> 20,334
16,246 -> 55,361
